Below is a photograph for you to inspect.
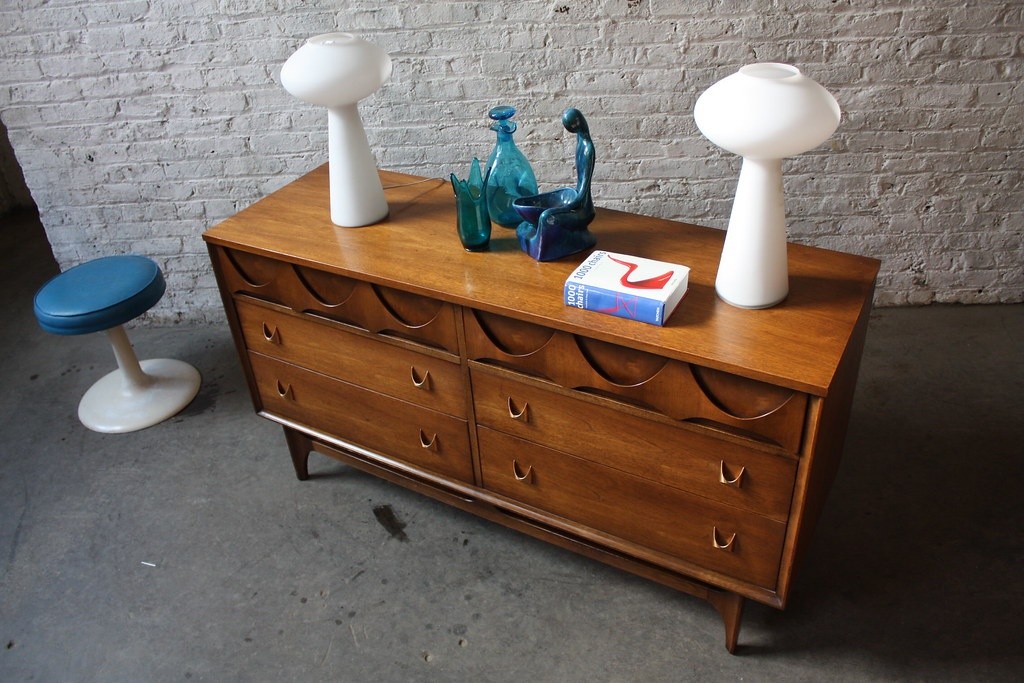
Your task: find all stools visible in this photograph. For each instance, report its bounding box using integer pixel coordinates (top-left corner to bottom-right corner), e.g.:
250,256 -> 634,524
33,253 -> 202,435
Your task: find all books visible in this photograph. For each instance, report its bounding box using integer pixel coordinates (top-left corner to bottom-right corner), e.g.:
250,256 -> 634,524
564,250 -> 691,326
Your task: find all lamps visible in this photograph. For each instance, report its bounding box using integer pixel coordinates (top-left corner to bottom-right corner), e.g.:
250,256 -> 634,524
280,32 -> 446,227
692,63 -> 840,312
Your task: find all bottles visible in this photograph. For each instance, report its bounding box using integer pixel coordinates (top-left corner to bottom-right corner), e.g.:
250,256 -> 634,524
482,106 -> 538,227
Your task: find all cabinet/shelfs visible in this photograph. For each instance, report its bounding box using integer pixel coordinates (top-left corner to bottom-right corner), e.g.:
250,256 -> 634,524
201,162 -> 881,655
